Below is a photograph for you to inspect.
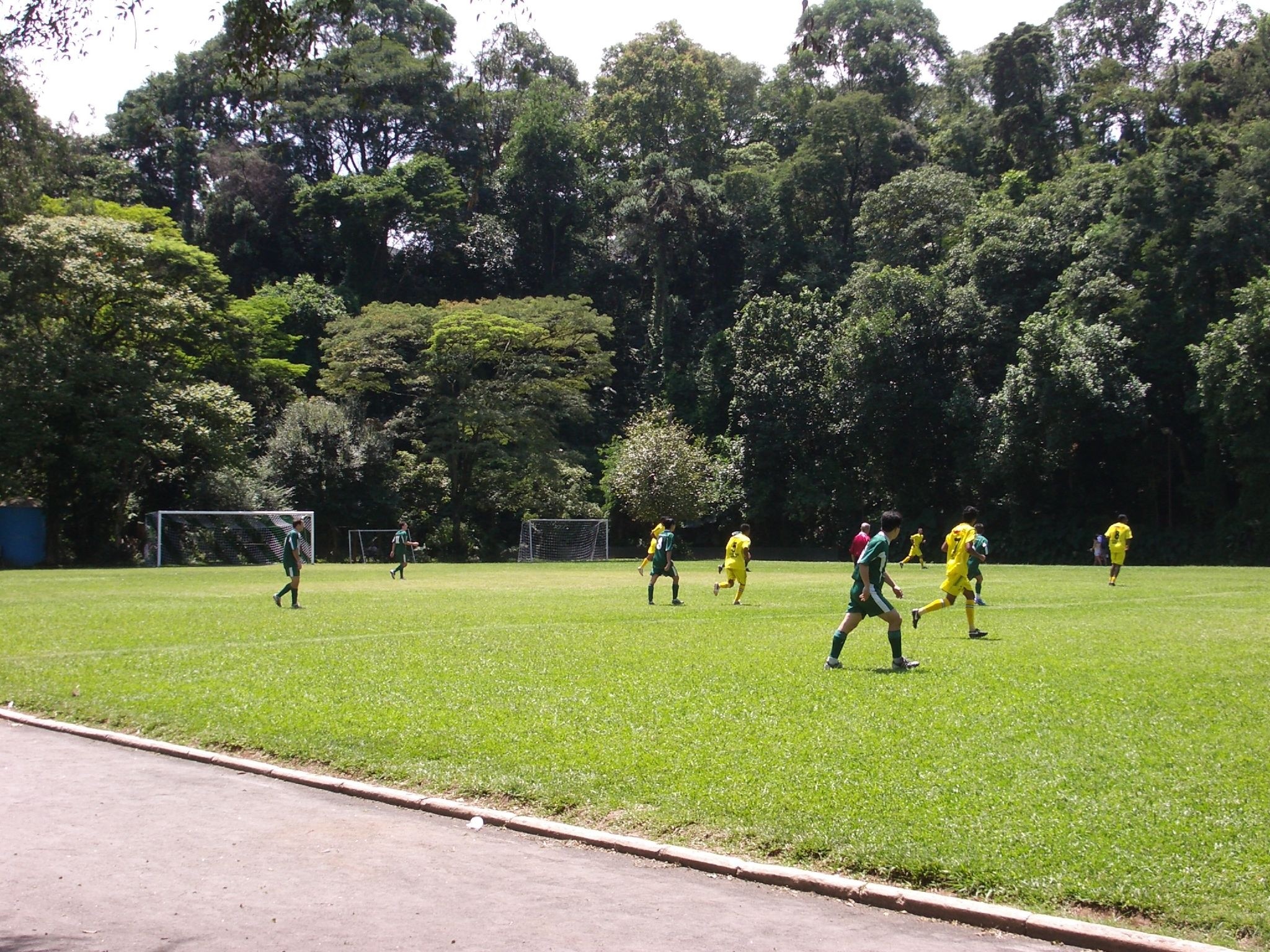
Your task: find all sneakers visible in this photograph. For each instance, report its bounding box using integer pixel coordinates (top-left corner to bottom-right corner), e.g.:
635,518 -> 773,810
733,601 -> 740,605
892,657 -> 920,670
824,661 -> 843,670
718,565 -> 721,574
649,602 -> 655,605
273,594 -> 282,608
291,604 -> 304,609
714,582 -> 719,596
975,598 -> 985,606
969,629 -> 988,638
912,608 -> 920,628
390,570 -> 395,580
746,568 -> 751,572
638,567 -> 643,576
672,600 -> 684,604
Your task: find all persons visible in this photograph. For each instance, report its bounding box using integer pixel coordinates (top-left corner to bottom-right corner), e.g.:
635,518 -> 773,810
390,521 -> 419,580
912,505 -> 988,638
849,523 -> 870,569
713,523 -> 752,606
273,517 -> 304,609
1104,514 -> 1133,586
637,516 -> 685,605
1086,533 -> 1109,566
823,510 -> 920,671
899,528 -> 929,569
967,524 -> 989,606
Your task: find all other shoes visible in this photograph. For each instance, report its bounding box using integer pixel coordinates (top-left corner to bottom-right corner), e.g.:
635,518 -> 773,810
900,561 -> 903,568
922,566 -> 927,569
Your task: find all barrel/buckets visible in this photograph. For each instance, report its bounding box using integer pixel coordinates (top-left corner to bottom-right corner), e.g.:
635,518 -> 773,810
0,505 -> 47,568
